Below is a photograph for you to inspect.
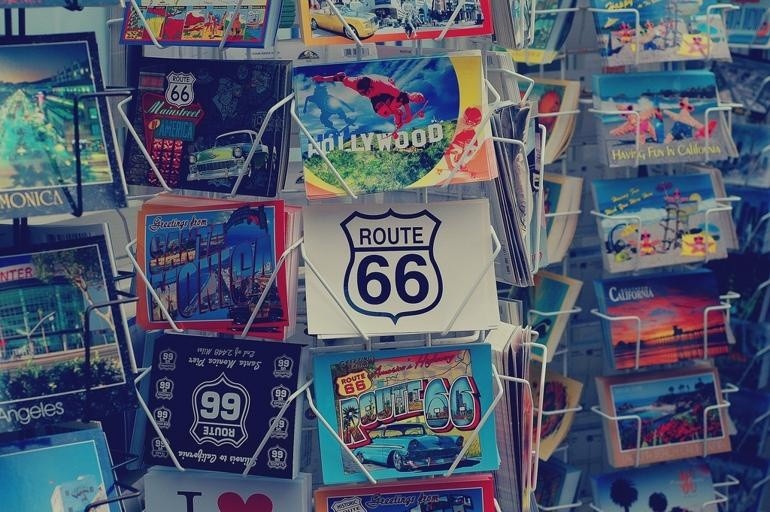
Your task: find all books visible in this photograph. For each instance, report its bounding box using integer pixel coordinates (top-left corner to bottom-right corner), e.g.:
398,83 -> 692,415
0,31 -> 139,512
292,1 -> 544,512
492,0 -> 769,512
121,1 -> 313,512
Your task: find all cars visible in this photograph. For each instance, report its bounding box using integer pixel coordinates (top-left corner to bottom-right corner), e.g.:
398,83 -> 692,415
311,5 -> 380,41
187,128 -> 274,187
353,420 -> 466,472
